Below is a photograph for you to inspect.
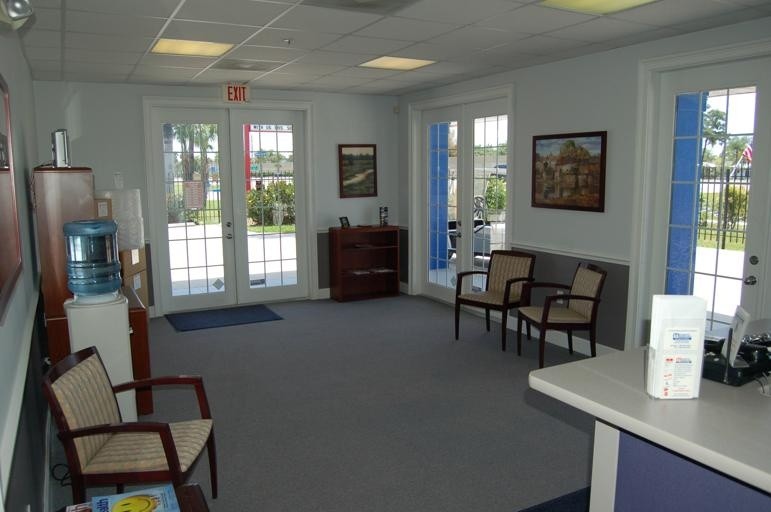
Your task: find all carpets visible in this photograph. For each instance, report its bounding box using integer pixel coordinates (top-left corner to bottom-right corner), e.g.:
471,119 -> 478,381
164,303 -> 282,330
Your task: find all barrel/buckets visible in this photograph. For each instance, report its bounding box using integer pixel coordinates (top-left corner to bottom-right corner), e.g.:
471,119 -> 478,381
62,220 -> 123,296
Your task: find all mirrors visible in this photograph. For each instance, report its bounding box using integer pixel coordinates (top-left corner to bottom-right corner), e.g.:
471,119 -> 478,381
0,71 -> 23,317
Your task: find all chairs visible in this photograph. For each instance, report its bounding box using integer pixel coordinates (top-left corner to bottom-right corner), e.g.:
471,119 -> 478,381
517,262 -> 607,368
41,346 -> 217,512
454,250 -> 536,351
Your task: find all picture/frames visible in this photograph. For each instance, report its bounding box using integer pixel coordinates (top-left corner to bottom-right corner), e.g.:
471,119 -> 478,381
340,217 -> 350,228
532,130 -> 607,213
339,144 -> 378,198
0,133 -> 10,171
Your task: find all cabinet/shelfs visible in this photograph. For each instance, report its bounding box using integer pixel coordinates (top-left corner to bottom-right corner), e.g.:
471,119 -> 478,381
33,167 -> 96,318
328,225 -> 401,303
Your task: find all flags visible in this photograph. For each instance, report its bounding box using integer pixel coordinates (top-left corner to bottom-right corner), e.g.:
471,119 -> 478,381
742,141 -> 753,162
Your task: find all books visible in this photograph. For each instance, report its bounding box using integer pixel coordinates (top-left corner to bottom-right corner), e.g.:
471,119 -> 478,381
66,485 -> 181,512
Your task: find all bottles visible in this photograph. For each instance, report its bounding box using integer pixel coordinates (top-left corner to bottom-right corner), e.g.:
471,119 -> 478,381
380,207 -> 388,227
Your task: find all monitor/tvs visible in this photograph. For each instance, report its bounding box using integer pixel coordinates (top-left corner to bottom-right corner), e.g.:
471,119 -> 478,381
51,129 -> 70,169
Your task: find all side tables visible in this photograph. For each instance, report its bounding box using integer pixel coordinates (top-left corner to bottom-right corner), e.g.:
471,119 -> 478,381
57,483 -> 210,512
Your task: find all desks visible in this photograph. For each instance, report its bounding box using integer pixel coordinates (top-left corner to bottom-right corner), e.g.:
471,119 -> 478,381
39,285 -> 154,415
528,333 -> 771,512
